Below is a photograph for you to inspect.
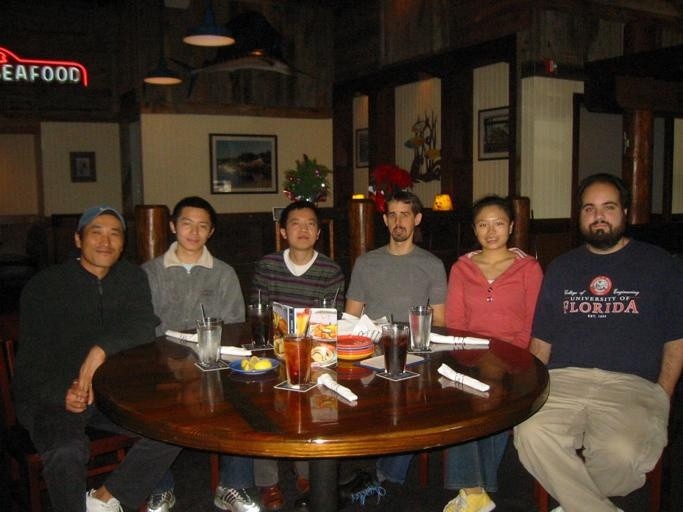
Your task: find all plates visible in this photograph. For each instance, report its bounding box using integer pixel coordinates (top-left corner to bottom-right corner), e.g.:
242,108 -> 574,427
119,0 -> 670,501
336,335 -> 375,361
229,357 -> 279,375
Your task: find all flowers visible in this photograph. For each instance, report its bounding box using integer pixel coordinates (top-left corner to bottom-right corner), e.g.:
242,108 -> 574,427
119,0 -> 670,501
283,153 -> 332,202
405,111 -> 442,182
367,164 -> 413,214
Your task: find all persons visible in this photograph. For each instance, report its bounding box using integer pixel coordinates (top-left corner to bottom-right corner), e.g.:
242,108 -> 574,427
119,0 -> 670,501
338,191 -> 448,512
15,204 -> 162,512
442,195 -> 545,512
250,202 -> 345,511
141,196 -> 261,512
513,173 -> 682,512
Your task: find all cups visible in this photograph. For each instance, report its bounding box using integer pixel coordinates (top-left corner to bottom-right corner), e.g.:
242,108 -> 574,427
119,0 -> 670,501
196,317 -> 222,364
283,335 -> 312,389
381,325 -> 409,375
409,306 -> 433,351
247,304 -> 271,347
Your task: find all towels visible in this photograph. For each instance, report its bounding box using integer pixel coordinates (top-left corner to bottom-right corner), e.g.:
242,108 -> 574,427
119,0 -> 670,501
317,373 -> 358,402
220,345 -> 253,356
438,363 -> 490,392
430,333 -> 489,345
164,330 -> 199,343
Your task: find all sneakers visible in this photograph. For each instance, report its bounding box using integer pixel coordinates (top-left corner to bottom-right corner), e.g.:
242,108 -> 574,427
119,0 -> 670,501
443,495 -> 461,512
295,473 -> 366,512
336,471 -> 386,510
456,488 -> 496,512
85,489 -> 124,512
141,487 -> 175,512
214,484 -> 263,512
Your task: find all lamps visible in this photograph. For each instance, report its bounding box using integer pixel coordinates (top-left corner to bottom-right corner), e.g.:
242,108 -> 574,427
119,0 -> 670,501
250,47 -> 266,59
144,76 -> 182,86
181,33 -> 236,49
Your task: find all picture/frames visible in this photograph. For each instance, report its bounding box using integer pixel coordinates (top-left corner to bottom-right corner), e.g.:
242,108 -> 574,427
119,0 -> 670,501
478,107 -> 509,161
69,152 -> 98,182
209,134 -> 279,193
356,129 -> 369,167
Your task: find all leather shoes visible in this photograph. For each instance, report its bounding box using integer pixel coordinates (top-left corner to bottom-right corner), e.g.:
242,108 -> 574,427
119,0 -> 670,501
295,476 -> 311,497
260,485 -> 286,511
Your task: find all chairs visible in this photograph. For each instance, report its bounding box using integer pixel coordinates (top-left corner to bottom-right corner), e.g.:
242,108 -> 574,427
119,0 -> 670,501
5,338 -> 154,511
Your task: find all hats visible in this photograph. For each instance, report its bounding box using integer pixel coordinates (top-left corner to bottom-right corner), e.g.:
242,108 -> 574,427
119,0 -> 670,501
78,207 -> 126,233
